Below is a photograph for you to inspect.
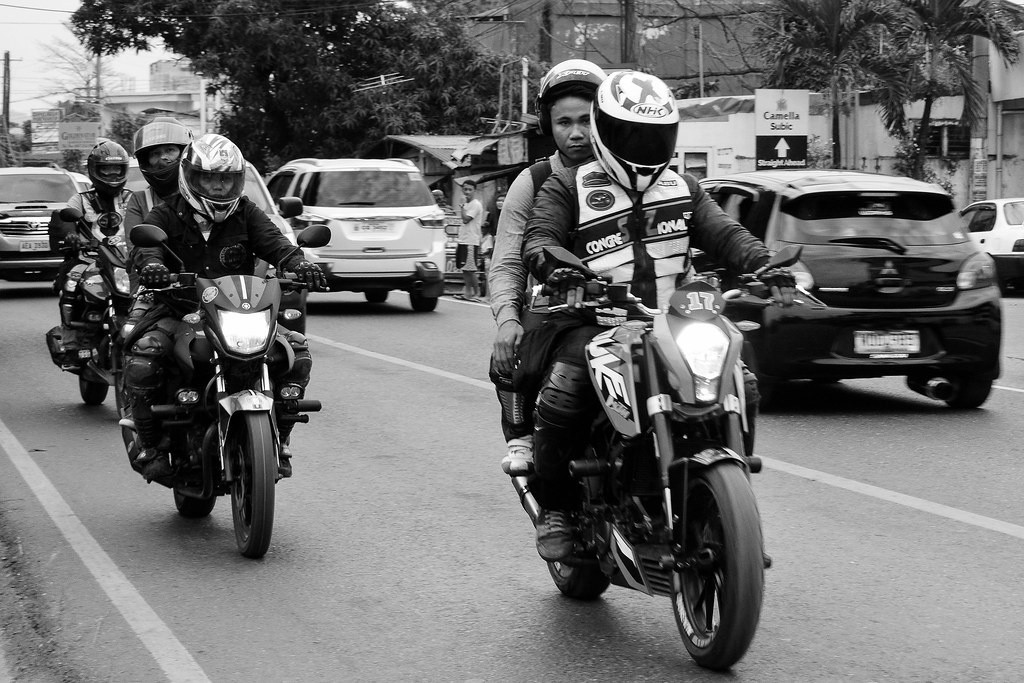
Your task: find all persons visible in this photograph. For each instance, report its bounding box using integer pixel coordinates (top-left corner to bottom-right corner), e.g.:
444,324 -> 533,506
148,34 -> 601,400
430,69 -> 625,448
484,193 -> 506,274
121,117 -> 329,477
453,179 -> 483,303
486,57 -> 798,567
57,139 -> 134,371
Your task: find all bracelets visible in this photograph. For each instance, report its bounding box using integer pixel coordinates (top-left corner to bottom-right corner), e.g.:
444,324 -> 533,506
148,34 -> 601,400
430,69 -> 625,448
459,204 -> 465,208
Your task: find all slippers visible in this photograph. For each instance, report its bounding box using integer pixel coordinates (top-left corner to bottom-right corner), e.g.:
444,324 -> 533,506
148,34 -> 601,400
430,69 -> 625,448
465,297 -> 482,302
452,294 -> 468,300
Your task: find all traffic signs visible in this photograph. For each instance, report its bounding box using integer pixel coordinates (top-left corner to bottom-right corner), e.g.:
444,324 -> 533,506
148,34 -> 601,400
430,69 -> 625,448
751,88 -> 810,172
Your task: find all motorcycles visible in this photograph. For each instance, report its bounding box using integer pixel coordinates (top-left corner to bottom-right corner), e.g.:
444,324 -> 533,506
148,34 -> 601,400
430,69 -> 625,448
111,223 -> 331,559
507,246 -> 829,672
44,205 -> 135,419
440,205 -> 489,298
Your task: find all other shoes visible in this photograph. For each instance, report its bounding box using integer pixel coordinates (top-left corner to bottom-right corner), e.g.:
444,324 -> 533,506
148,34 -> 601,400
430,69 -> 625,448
60,341 -> 80,370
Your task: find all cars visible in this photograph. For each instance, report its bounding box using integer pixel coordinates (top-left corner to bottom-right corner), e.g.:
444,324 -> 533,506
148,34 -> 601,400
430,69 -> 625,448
957,196 -> 1024,292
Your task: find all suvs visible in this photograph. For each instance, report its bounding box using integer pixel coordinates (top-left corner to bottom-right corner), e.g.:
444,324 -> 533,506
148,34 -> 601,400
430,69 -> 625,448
124,154 -> 308,336
690,169 -> 1002,416
264,156 -> 449,314
0,161 -> 93,281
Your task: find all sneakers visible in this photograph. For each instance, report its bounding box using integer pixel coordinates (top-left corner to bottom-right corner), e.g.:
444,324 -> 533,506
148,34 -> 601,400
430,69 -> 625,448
279,435 -> 293,478
134,436 -> 171,481
534,506 -> 574,562
502,433 -> 534,478
762,551 -> 771,568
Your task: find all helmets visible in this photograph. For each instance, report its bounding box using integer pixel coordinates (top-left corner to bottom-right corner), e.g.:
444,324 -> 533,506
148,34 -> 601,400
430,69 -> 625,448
535,58 -> 608,134
131,118 -> 194,197
87,139 -> 130,196
589,70 -> 680,193
178,135 -> 246,223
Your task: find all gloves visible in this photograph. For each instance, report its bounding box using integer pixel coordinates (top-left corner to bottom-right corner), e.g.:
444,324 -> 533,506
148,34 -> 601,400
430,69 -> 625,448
140,262 -> 171,289
290,261 -> 327,292
63,233 -> 81,251
